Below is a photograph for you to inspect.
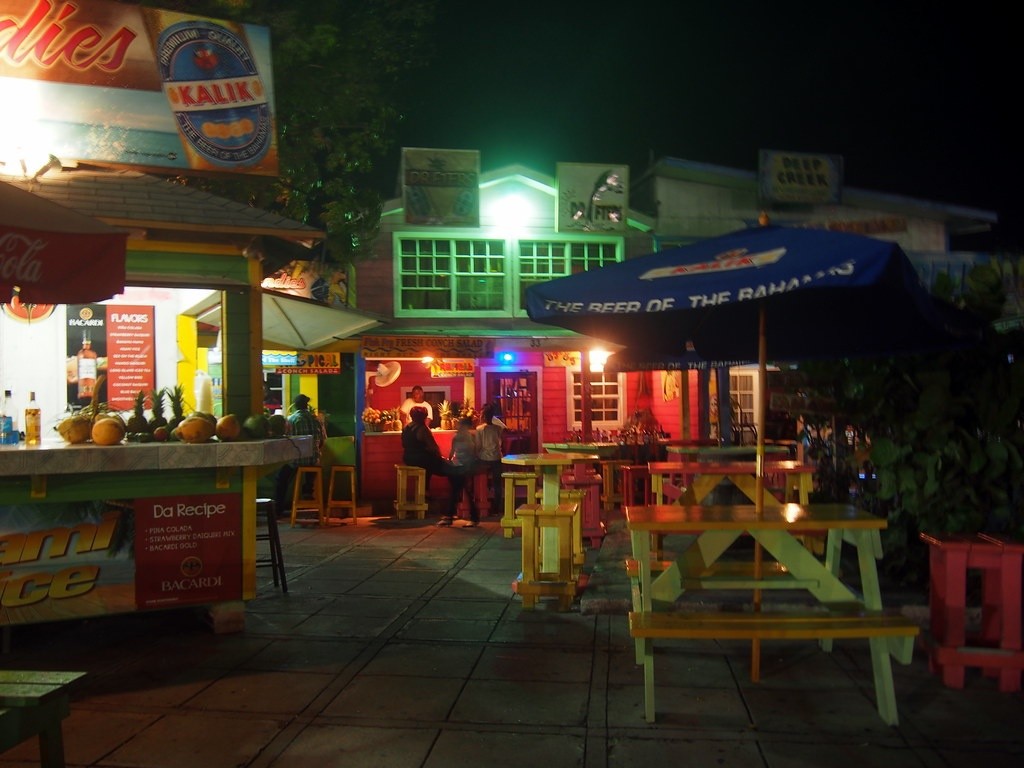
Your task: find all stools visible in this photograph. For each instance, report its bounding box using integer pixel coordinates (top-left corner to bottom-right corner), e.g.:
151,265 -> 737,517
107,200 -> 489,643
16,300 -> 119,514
456,459 -> 651,612
393,463 -> 428,519
919,531 -> 1024,693
256,498 -> 288,593
291,467 -> 324,527
326,466 -> 357,525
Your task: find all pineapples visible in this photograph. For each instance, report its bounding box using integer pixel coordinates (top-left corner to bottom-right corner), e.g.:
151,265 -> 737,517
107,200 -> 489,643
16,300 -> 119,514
391,407 -> 402,431
438,400 -> 453,430
126,388 -> 185,433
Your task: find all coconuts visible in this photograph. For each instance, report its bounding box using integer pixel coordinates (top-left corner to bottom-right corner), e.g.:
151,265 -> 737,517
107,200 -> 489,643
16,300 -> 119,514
175,411 -> 287,443
55,411 -> 127,446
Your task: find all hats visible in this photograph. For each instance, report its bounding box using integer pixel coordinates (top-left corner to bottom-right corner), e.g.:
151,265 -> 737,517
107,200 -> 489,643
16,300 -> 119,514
294,394 -> 310,406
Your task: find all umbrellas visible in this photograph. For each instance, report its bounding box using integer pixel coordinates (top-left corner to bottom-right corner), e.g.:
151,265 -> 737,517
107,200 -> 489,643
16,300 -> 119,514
523,211 -> 927,684
0,180 -> 128,304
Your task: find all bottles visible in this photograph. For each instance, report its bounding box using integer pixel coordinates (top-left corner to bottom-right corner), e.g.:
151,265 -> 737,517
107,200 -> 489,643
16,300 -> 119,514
572,427 -> 576,443
78,330 -> 97,403
593,427 -> 608,442
578,429 -> 582,444
0,390 -> 19,445
618,427 -> 638,446
643,424 -> 671,444
25,392 -> 41,445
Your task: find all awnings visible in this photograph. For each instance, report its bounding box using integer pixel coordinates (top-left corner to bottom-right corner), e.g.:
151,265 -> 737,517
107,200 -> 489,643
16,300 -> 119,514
195,289 -> 392,350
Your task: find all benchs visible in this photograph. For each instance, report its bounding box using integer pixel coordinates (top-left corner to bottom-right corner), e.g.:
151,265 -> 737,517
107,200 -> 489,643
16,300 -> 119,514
629,611 -> 921,638
624,558 -> 791,576
0,670 -> 89,768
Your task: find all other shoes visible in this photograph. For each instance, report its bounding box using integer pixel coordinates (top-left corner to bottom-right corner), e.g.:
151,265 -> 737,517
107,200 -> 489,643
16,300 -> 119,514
437,518 -> 453,525
464,521 -> 480,527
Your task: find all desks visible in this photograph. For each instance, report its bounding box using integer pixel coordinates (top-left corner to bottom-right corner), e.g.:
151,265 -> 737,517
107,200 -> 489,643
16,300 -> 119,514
500,439 -> 916,727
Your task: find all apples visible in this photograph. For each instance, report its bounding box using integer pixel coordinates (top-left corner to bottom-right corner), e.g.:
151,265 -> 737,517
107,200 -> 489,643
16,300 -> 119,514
362,407 -> 381,424
153,427 -> 167,441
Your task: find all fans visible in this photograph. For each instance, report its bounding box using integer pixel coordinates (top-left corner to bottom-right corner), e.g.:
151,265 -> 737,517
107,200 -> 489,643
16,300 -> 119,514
366,362 -> 401,395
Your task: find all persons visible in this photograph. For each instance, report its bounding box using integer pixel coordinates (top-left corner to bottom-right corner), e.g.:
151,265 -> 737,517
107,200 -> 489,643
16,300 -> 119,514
400,386 -> 433,429
273,395 -> 327,520
401,403 -> 508,527
